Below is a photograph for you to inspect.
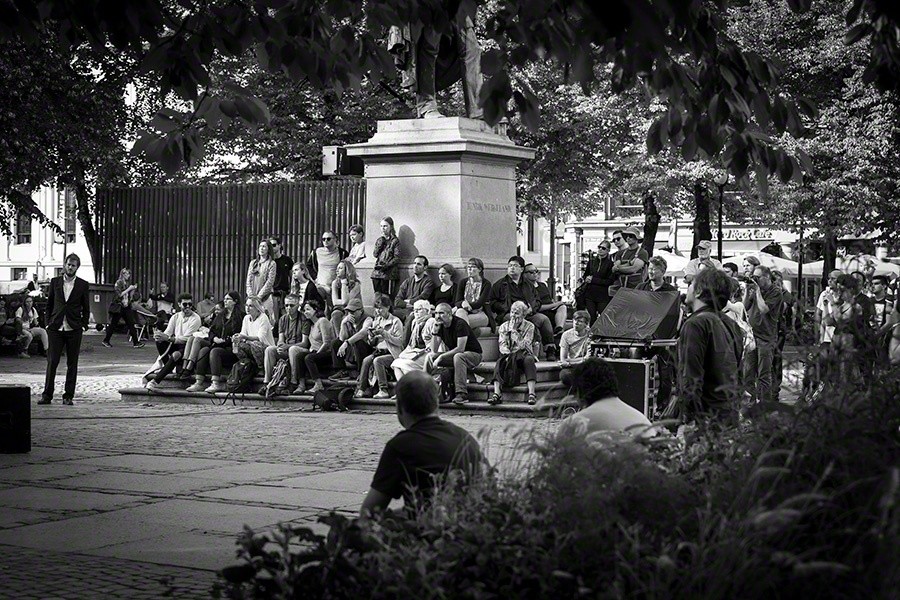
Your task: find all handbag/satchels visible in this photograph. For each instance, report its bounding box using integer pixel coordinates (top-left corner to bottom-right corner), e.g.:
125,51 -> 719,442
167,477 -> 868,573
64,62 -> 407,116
574,282 -> 589,310
737,306 -> 756,353
108,302 -> 122,315
143,342 -> 173,383
1,317 -> 19,340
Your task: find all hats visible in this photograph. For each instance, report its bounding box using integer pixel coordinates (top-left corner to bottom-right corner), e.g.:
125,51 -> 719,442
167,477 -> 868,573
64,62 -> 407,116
344,299 -> 363,311
695,240 -> 712,249
622,227 -> 643,243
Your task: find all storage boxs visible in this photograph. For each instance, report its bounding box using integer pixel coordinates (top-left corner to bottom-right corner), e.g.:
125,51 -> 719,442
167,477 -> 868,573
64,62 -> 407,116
605,355 -> 656,421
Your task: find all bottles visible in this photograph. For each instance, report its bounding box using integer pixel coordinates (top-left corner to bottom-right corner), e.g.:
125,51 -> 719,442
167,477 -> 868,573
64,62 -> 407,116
233,343 -> 238,353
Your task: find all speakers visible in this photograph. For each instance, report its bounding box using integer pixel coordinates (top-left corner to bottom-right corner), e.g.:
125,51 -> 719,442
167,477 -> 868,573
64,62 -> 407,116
0,384 -> 31,454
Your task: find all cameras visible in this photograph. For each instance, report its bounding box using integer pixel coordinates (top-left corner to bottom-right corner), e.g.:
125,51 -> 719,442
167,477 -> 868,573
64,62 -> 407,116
744,276 -> 759,283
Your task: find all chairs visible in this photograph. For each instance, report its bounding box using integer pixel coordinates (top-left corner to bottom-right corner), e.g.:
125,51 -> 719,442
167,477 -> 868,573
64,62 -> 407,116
127,308 -> 156,343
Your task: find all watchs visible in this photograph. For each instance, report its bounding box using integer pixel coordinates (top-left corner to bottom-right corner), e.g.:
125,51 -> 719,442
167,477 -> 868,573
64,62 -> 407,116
167,336 -> 171,343
345,338 -> 350,347
245,238 -> 276,325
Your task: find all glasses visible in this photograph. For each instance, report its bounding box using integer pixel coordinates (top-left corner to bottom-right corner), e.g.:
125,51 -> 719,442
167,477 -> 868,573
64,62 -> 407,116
272,244 -> 279,247
322,237 -> 334,241
624,234 -> 634,238
612,237 -> 622,242
284,303 -> 298,307
182,302 -> 193,306
598,246 -> 609,251
525,269 -> 538,273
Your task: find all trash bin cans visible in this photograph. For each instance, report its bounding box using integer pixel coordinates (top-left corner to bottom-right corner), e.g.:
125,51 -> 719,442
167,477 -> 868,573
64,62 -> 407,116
89,284 -> 115,331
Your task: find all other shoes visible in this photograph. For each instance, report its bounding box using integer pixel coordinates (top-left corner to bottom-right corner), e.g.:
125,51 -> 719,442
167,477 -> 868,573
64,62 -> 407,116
134,342 -> 144,348
102,340 -> 112,347
62,399 -> 73,404
554,329 -> 564,339
18,351 -> 30,358
145,371 -> 537,404
547,351 -> 556,360
38,396 -> 51,403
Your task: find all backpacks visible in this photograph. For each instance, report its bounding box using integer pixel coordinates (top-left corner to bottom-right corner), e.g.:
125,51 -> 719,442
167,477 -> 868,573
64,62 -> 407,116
227,358 -> 258,393
267,358 -> 293,395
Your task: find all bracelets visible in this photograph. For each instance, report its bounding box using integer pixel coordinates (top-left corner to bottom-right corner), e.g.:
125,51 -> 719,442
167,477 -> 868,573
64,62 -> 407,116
432,332 -> 438,336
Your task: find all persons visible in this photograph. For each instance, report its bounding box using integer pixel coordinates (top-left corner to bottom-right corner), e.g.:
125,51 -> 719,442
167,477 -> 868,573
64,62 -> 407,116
128,216 -> 900,448
355,370 -> 502,522
36,251 -> 90,405
258,293 -> 311,397
485,300 -> 538,405
422,302 -> 484,402
558,310 -> 593,394
100,267 -> 145,349
555,357 -> 656,469
0,274 -> 48,359
454,257 -> 496,334
328,298 -> 375,382
673,267 -> 743,431
